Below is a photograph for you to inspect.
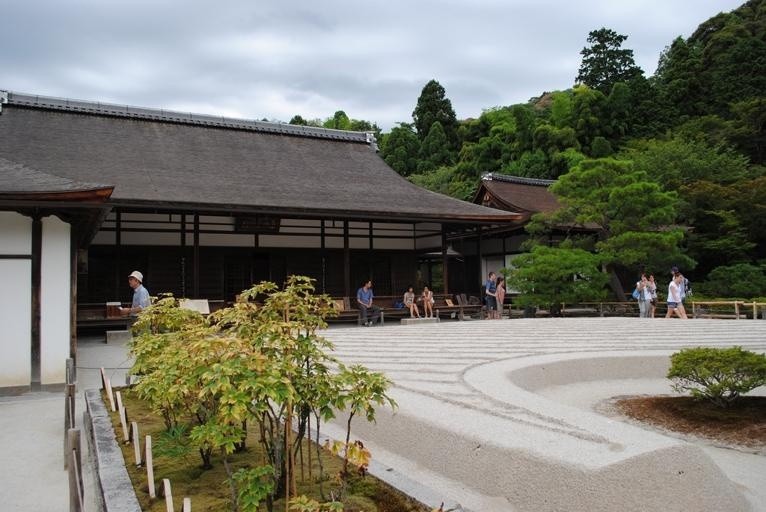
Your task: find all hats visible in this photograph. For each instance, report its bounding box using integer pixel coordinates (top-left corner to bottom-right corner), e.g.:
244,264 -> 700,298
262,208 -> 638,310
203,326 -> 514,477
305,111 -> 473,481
671,267 -> 678,271
129,270 -> 143,283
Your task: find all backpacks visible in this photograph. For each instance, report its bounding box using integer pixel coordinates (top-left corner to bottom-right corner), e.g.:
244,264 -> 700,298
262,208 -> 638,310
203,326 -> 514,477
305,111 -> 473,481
684,279 -> 693,295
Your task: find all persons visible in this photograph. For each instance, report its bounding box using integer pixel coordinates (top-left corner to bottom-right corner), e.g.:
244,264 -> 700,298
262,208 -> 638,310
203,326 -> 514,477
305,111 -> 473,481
670,267 -> 689,319
484,271 -> 497,320
636,271 -> 654,318
664,273 -> 689,319
119,269 -> 153,346
493,276 -> 505,319
355,279 -> 380,327
420,286 -> 435,318
402,285 -> 422,318
647,273 -> 658,318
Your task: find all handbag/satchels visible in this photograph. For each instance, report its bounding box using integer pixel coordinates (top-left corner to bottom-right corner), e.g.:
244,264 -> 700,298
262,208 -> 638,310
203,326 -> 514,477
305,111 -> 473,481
632,289 -> 641,300
393,302 -> 405,307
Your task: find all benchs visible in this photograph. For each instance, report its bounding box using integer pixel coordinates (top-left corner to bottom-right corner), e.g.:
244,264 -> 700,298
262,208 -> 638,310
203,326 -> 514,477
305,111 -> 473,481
77,302 -> 146,331
285,298 -> 361,327
368,297 -> 482,321
151,299 -> 238,328
